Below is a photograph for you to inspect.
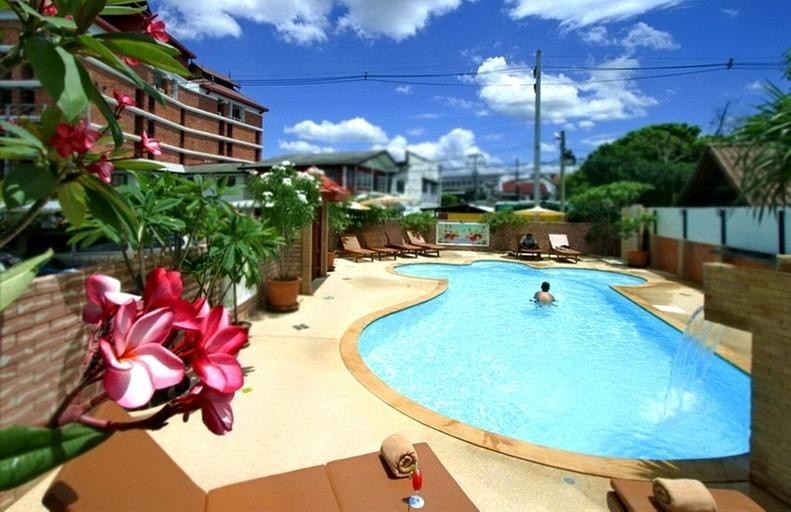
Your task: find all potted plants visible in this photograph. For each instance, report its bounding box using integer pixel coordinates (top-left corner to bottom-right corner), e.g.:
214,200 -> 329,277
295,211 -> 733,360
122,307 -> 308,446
614,211 -> 657,268
199,204 -> 287,351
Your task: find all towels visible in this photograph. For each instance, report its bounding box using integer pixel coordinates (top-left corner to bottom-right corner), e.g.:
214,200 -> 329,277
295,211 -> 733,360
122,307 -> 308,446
652,477 -> 719,512
379,434 -> 417,479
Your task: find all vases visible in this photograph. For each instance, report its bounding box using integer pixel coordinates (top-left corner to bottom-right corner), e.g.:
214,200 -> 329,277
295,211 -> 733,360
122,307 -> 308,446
263,272 -> 299,312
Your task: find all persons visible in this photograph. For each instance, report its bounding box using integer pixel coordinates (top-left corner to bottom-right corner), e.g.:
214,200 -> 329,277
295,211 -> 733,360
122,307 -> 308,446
519,233 -> 538,249
534,282 -> 555,306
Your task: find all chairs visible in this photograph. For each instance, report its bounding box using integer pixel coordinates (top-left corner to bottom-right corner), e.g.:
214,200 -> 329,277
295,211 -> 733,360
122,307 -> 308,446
41,396 -> 480,511
611,476 -> 766,512
341,228 -> 446,262
509,233 -> 543,260
546,233 -> 582,264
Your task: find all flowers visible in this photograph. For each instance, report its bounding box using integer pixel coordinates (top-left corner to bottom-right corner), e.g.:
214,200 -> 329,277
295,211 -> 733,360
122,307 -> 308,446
247,160 -> 323,279
0,0 -> 248,488
